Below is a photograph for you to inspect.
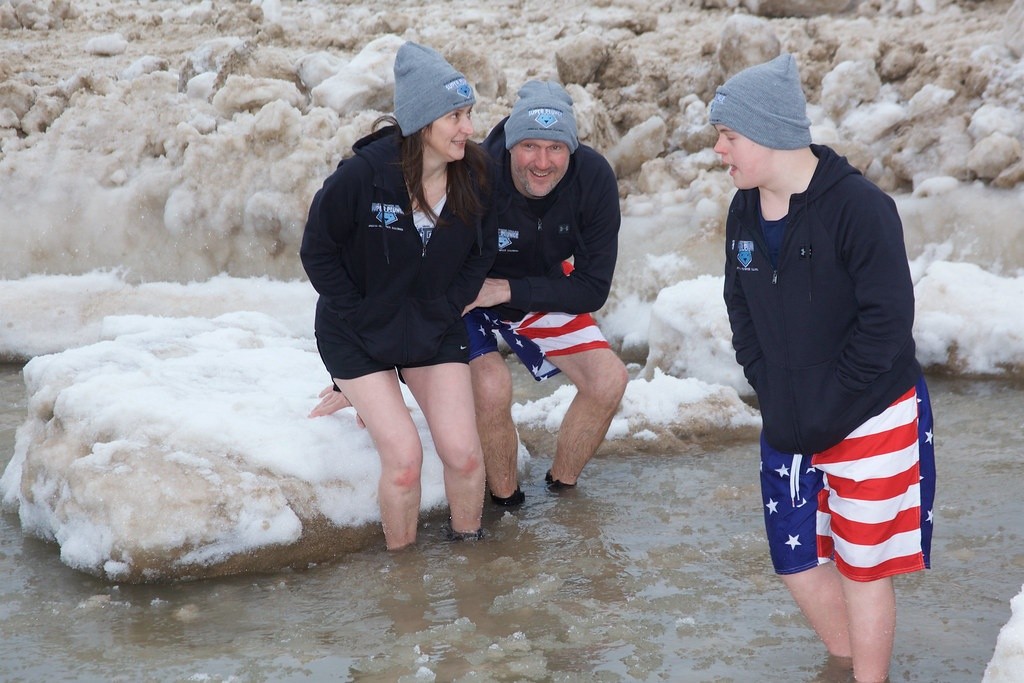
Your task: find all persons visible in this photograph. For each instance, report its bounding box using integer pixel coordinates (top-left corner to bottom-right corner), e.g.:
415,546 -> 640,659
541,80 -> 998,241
299,43 -> 497,551
710,50 -> 937,681
310,79 -> 627,518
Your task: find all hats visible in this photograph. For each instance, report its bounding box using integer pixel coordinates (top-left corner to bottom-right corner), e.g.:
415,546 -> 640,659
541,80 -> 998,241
394,42 -> 477,137
709,52 -> 813,149
504,80 -> 580,155
